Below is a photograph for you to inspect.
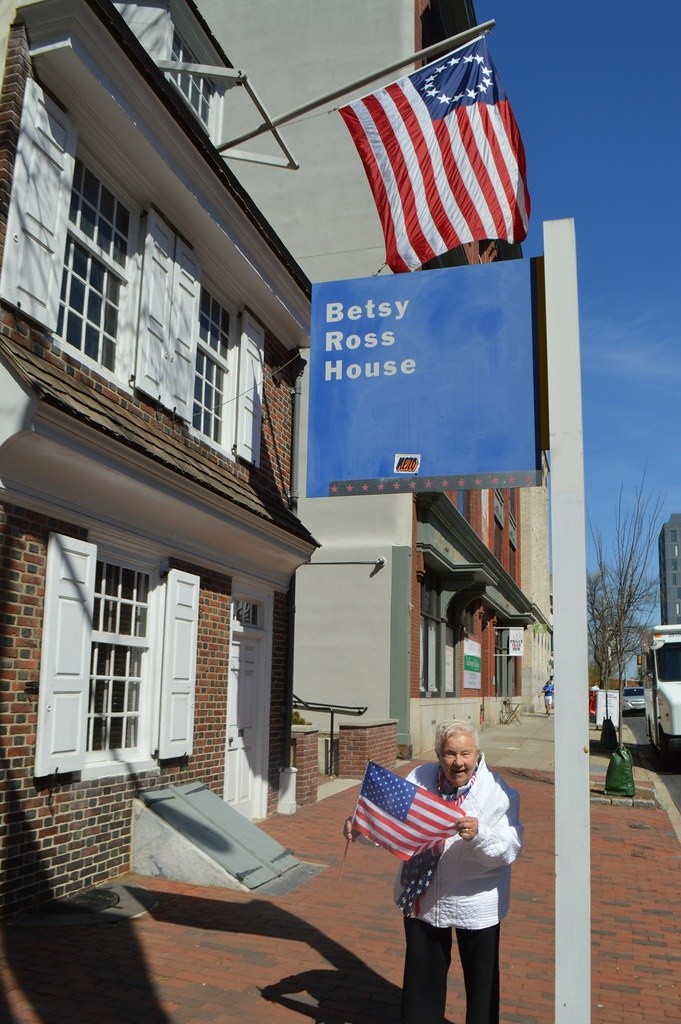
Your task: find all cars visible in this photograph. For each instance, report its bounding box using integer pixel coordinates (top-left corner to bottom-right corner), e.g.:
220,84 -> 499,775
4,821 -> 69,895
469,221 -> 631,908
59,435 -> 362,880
622,688 -> 646,716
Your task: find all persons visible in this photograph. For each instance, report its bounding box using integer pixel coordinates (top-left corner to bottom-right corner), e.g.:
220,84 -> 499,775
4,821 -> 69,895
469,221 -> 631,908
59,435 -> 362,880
541,679 -> 555,716
345,719 -> 523,1023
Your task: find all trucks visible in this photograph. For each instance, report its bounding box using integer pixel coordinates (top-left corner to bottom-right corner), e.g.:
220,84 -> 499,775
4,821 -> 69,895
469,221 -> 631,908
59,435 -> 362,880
641,624 -> 681,774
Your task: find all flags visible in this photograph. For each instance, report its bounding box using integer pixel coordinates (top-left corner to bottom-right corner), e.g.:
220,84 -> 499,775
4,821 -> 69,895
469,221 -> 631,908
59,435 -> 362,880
337,27 -> 532,275
350,759 -> 466,865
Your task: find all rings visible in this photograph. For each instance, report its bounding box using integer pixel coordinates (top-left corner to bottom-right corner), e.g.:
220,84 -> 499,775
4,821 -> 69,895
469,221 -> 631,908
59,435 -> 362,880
466,829 -> 468,831
